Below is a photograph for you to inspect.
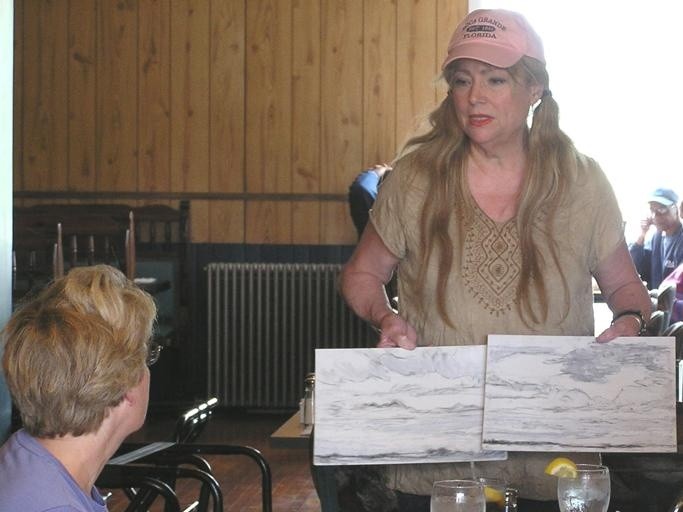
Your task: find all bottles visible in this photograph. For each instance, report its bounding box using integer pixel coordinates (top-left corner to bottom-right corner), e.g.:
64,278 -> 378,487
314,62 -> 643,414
503,487 -> 519,512
303,372 -> 315,426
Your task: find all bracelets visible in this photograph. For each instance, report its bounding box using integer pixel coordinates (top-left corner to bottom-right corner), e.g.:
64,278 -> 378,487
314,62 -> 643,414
610,308 -> 648,337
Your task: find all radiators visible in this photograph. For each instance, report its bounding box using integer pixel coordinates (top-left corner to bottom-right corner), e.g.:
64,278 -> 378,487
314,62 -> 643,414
202,261 -> 397,422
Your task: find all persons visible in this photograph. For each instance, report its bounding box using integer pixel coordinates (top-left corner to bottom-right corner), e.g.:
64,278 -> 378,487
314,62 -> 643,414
0,261 -> 158,512
652,257 -> 683,327
631,186 -> 683,287
333,6 -> 658,504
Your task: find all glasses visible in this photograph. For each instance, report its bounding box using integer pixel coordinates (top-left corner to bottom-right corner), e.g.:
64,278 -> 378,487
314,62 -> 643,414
137,341 -> 163,370
649,203 -> 673,217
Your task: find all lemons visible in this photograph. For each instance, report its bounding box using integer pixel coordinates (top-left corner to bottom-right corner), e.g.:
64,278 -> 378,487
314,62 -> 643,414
544,457 -> 577,479
484,487 -> 503,502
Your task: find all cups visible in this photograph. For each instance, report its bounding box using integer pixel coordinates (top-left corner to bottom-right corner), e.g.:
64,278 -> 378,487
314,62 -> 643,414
431,480 -> 486,512
556,464 -> 611,512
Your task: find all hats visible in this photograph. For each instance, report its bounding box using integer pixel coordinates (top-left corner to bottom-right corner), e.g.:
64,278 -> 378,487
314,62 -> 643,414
647,186 -> 678,210
439,8 -> 546,70
